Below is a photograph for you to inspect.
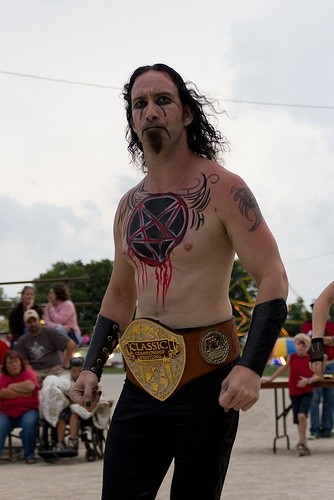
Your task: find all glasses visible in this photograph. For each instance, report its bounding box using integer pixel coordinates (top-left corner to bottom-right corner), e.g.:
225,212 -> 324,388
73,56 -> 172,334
70,356 -> 84,365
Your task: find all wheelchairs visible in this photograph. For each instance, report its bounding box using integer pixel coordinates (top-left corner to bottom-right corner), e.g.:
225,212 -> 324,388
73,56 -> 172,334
40,419 -> 105,463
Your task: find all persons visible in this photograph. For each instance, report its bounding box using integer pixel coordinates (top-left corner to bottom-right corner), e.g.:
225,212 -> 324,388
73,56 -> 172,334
307,278 -> 334,376
302,298 -> 334,441
260,333 -> 328,456
0,281 -> 107,464
70,63 -> 290,500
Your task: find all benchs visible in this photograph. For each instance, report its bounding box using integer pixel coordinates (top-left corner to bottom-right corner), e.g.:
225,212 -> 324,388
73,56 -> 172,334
0,277 -> 94,350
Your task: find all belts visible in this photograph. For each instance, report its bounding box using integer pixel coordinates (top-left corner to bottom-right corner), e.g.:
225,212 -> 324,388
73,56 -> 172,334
118,316 -> 242,403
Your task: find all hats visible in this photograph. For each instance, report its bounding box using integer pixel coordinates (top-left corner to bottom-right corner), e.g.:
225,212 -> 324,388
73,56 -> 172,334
23,309 -> 41,324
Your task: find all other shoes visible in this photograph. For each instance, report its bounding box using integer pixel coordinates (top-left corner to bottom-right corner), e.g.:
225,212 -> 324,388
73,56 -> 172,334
0,436 -> 80,464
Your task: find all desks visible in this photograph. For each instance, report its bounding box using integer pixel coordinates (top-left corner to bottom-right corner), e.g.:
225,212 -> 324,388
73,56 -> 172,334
260,379 -> 334,454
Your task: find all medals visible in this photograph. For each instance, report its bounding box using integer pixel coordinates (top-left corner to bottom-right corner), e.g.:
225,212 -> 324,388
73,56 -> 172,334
119,316 -> 241,402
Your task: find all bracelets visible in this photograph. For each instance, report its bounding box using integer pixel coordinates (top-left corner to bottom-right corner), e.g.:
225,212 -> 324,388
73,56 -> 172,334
307,377 -> 312,384
